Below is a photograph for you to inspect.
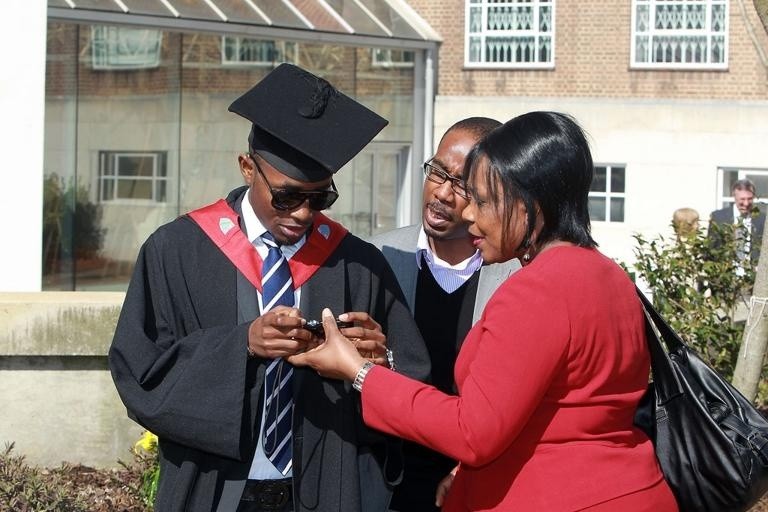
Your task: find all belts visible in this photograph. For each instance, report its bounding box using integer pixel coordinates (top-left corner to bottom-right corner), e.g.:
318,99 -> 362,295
238,474 -> 293,509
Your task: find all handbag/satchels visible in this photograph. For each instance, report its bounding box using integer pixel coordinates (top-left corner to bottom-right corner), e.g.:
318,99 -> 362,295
626,276 -> 768,512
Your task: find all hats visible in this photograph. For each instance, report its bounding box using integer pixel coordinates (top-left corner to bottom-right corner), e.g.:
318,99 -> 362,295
228,63 -> 391,186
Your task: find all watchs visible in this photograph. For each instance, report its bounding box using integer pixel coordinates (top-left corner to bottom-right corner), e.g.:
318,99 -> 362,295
353,361 -> 375,392
386,349 -> 395,371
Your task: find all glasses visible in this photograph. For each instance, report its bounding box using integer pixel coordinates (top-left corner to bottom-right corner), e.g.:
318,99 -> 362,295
248,156 -> 341,212
422,156 -> 475,201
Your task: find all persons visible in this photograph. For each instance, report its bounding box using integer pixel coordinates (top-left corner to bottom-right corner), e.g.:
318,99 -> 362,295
110,64 -> 431,512
284,110 -> 680,512
671,180 -> 767,290
362,117 -> 523,512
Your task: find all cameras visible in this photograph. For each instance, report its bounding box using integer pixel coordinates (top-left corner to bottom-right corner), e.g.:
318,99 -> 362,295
303,320 -> 354,342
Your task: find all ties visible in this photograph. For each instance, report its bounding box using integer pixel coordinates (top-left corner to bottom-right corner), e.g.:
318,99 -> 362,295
733,217 -> 746,267
258,230 -> 298,478
